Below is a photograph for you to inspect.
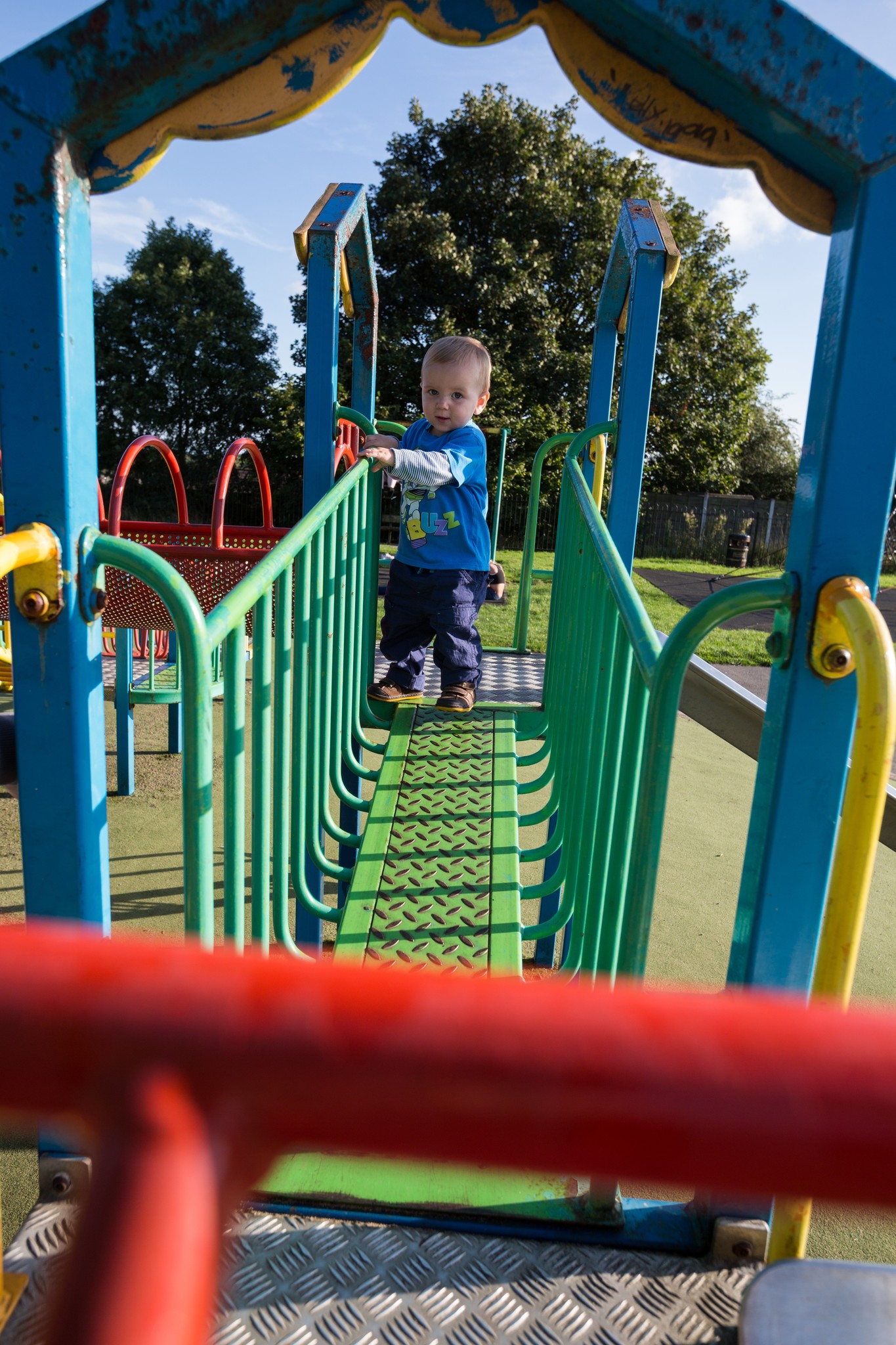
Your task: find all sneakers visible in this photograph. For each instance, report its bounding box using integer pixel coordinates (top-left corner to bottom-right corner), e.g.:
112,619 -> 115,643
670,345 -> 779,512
435,682 -> 476,712
367,677 -> 424,702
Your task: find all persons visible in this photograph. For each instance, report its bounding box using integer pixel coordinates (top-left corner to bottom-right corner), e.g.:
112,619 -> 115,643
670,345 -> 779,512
357,336 -> 491,712
483,562 -> 506,600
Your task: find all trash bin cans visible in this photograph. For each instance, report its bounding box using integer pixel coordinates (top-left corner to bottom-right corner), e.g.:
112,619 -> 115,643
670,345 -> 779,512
726,533 -> 750,569
